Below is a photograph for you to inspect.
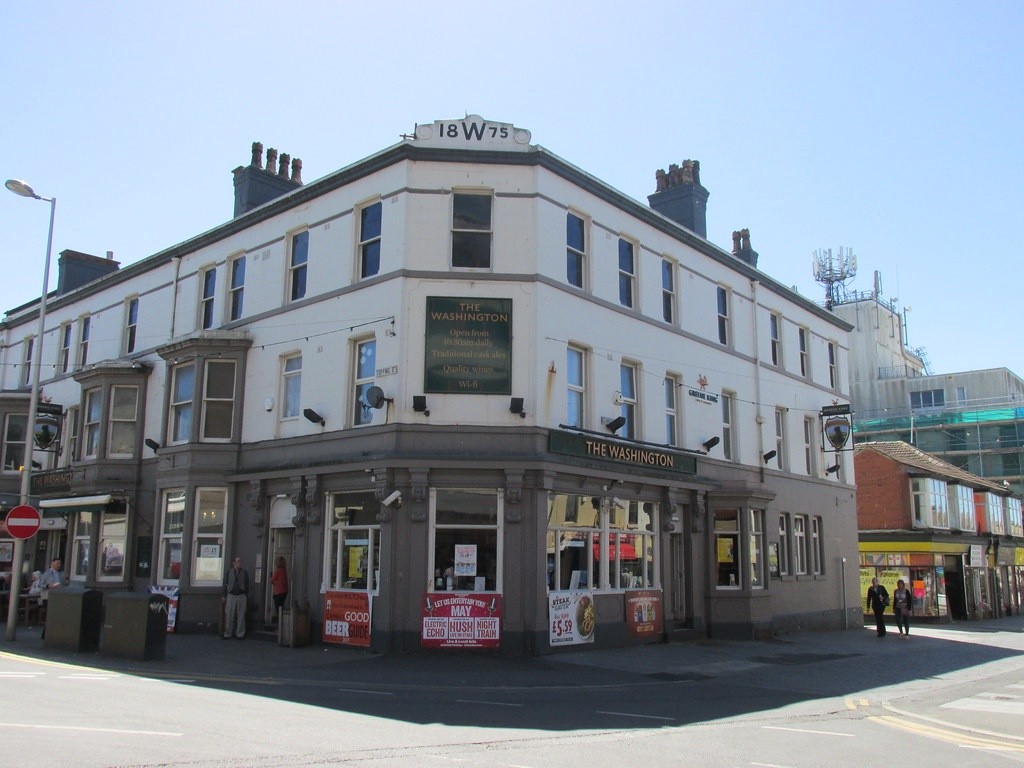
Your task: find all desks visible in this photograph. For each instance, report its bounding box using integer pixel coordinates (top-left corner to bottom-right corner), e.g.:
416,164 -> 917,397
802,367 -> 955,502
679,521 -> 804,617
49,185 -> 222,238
18,594 -> 41,627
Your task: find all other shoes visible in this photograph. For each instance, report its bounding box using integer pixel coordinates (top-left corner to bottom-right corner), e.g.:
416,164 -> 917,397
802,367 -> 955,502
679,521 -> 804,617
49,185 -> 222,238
902,635 -> 909,639
898,632 -> 904,639
222,636 -> 230,641
238,637 -> 245,640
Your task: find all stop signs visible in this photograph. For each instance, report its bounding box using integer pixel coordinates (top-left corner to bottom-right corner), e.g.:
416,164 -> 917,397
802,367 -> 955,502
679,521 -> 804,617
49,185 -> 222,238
5,505 -> 42,541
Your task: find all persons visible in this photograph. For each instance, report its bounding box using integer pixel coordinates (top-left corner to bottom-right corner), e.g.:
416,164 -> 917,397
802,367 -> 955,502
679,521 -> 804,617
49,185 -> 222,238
222,555 -> 250,640
893,580 -> 913,640
270,556 -> 288,633
866,577 -> 890,638
29,557 -> 68,638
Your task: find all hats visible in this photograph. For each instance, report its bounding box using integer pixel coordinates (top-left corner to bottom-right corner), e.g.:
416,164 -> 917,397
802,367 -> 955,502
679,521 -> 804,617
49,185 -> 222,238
31,571 -> 42,580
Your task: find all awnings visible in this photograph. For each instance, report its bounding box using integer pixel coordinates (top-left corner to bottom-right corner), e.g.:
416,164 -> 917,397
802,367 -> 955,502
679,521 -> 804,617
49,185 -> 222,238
39,494 -> 125,511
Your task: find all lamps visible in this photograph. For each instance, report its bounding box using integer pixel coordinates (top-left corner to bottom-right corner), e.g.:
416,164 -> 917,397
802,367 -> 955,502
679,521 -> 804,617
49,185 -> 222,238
703,437 -> 719,452
413,396 -> 429,416
304,408 -> 324,427
510,398 -> 525,418
607,416 -> 625,434
145,439 -> 160,454
764,451 -> 776,463
826,465 -> 840,476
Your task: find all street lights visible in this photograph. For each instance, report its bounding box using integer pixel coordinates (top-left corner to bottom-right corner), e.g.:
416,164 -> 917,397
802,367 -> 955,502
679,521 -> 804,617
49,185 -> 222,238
4,178 -> 57,644
